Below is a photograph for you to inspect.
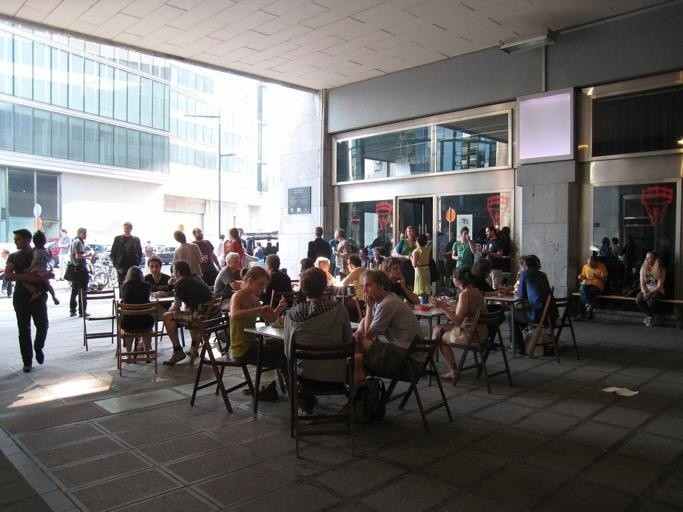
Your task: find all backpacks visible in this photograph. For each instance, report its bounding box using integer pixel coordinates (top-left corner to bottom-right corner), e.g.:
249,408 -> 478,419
349,376 -> 388,427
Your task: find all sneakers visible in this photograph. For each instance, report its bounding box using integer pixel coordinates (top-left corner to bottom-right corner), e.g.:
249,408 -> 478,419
52,296 -> 58,305
22,360 -> 32,374
29,291 -> 41,301
33,342 -> 44,365
78,311 -> 88,317
586,305 -> 594,313
297,408 -> 316,426
505,343 -> 525,353
186,350 -> 199,360
163,350 -> 186,366
70,312 -> 75,316
574,314 -> 584,322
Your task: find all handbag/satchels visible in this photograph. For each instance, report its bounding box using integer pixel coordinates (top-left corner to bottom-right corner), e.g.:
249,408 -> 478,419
63,260 -> 85,285
513,300 -> 532,324
241,380 -> 279,403
427,249 -> 440,285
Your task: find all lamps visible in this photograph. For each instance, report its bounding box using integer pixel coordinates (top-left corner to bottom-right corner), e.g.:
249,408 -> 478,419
499,28 -> 556,56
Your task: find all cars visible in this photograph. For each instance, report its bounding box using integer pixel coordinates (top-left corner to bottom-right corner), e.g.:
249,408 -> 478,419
153,246 -> 175,264
88,244 -> 112,255
91,256 -> 109,266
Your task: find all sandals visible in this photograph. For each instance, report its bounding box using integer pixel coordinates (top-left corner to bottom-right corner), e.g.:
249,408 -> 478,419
438,369 -> 461,381
645,314 -> 655,329
643,313 -> 649,324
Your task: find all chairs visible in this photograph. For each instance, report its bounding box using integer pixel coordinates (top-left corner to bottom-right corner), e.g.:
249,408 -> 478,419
450,309 -> 512,394
191,312 -> 255,414
269,288 -> 296,316
114,300 -> 159,377
288,336 -> 356,458
190,296 -> 224,365
80,289 -> 115,351
526,286 -> 579,364
382,328 -> 454,432
354,296 -> 366,322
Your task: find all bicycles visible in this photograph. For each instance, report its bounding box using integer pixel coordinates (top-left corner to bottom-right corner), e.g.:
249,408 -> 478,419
94,265 -> 119,287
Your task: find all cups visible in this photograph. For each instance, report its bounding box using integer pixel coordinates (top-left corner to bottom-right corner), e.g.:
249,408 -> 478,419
483,244 -> 488,250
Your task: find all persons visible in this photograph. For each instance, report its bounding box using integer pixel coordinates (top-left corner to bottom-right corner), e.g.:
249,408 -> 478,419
347,269 -> 428,400
110,222 -> 559,392
64,229 -> 94,317
577,237 -> 668,329
4,229 -> 48,372
59,229 -> 70,282
22,230 -> 60,305
0,249 -> 13,298
283,268 -> 353,424
